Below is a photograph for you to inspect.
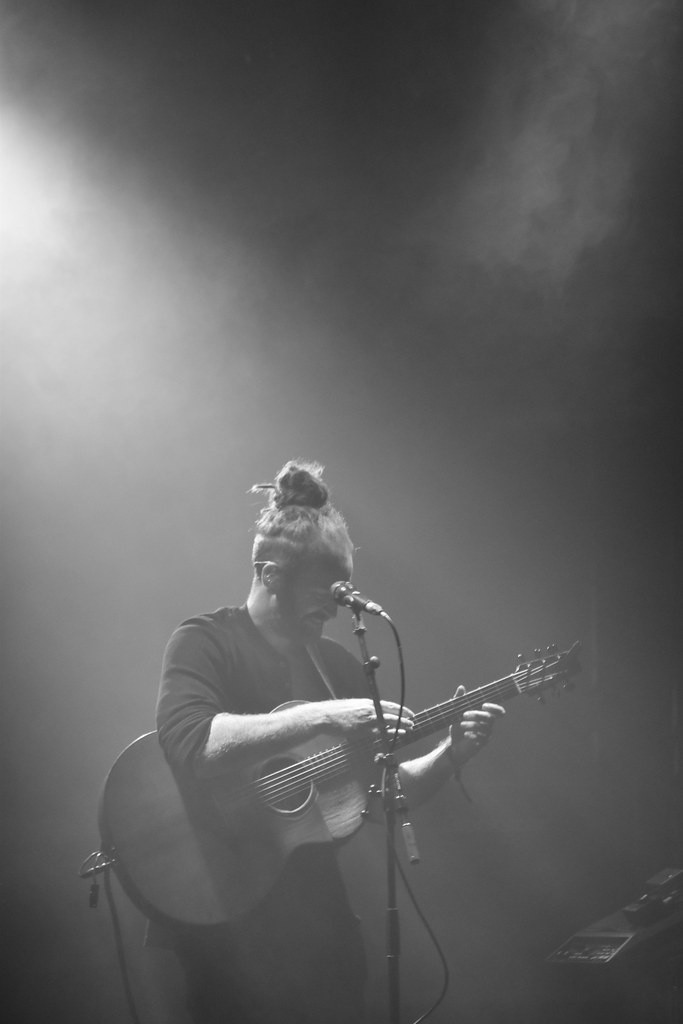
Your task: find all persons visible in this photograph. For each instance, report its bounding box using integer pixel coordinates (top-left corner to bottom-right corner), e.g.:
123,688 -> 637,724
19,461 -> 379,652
158,460 -> 506,1024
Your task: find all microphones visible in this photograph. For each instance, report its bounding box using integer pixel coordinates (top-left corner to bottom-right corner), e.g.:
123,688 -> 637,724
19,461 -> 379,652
329,581 -> 383,616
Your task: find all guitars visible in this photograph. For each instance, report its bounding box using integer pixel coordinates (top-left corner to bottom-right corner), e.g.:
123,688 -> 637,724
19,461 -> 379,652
97,638 -> 587,932
